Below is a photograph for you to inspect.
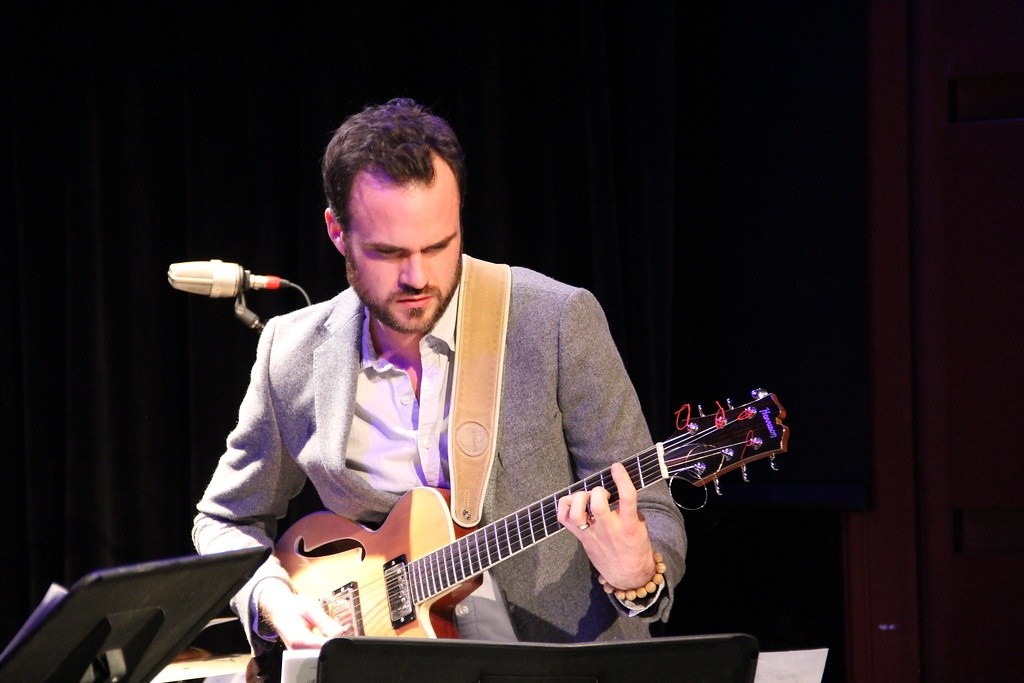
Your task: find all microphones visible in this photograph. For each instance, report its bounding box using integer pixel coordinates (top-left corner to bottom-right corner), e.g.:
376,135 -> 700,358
168,259 -> 289,297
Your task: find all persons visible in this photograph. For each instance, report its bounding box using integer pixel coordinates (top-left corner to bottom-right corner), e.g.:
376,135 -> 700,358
190,98 -> 689,651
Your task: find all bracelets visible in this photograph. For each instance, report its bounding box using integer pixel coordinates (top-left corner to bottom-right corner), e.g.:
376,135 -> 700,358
598,550 -> 667,601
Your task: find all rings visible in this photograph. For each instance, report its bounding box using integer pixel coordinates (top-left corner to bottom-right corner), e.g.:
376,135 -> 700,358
577,521 -> 590,531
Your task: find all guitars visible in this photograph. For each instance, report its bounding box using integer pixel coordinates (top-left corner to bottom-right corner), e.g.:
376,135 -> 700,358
246,383 -> 794,683
